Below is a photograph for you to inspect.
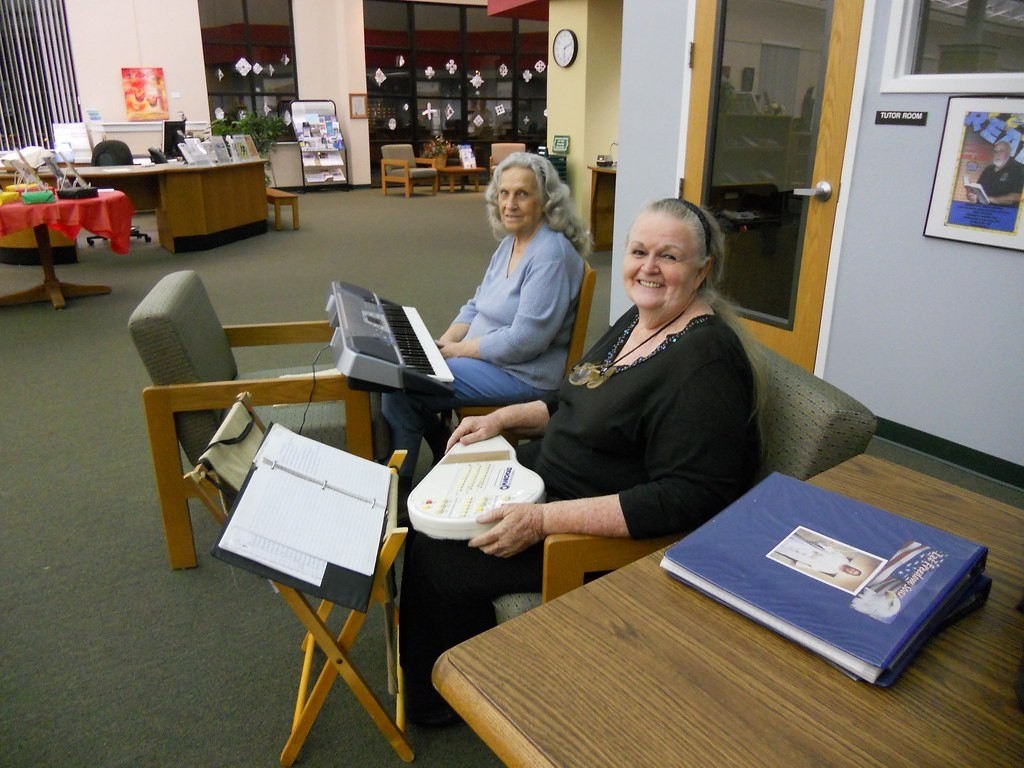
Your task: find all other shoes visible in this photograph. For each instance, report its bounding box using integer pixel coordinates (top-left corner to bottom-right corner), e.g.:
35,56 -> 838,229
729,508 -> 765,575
411,707 -> 460,730
398,491 -> 411,520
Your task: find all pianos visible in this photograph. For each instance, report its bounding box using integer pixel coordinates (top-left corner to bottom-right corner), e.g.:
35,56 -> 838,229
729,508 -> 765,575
325,277 -> 458,399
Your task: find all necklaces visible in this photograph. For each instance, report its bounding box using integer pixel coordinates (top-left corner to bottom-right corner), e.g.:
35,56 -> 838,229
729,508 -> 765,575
586,308 -> 685,390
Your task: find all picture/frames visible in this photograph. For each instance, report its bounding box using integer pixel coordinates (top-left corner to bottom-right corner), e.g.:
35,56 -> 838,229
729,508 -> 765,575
921,93 -> 1024,251
550,135 -> 572,153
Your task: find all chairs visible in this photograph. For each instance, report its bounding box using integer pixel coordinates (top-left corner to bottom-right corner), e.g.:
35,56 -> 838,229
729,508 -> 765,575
493,288 -> 875,628
381,144 -> 439,198
436,258 -> 600,441
490,142 -> 526,183
148,147 -> 168,164
84,140 -> 152,246
129,270 -> 378,572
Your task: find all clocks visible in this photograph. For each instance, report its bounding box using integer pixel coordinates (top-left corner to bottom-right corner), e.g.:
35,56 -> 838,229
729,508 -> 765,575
553,29 -> 579,69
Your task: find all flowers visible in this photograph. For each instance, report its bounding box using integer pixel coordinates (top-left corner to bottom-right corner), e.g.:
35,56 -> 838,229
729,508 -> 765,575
422,136 -> 456,158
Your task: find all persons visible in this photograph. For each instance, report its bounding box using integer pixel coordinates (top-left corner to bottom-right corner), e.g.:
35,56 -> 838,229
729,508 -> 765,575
399,197 -> 770,725
966,141 -> 1024,210
381,151 -> 597,517
776,533 -> 861,576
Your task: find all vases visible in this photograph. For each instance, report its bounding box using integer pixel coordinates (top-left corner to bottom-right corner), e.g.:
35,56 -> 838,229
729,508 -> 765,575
431,155 -> 447,168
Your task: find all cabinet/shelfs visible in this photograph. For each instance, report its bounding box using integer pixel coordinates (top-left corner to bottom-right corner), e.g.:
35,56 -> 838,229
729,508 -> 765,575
290,99 -> 350,194
715,112 -> 790,193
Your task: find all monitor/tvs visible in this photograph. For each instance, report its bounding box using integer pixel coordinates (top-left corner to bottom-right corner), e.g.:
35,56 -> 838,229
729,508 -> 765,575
161,120 -> 187,160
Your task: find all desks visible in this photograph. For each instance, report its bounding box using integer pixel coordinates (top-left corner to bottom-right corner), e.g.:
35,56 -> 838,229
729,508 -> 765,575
426,452 -> 1024,768
0,187 -> 130,312
431,166 -> 486,193
587,163 -> 617,252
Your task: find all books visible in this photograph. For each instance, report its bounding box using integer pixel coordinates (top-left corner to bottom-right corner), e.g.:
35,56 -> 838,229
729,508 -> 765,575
659,470 -> 993,687
963,175 -> 990,205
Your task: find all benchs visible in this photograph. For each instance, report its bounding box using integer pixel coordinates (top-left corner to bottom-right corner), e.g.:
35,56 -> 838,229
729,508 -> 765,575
265,186 -> 300,231
